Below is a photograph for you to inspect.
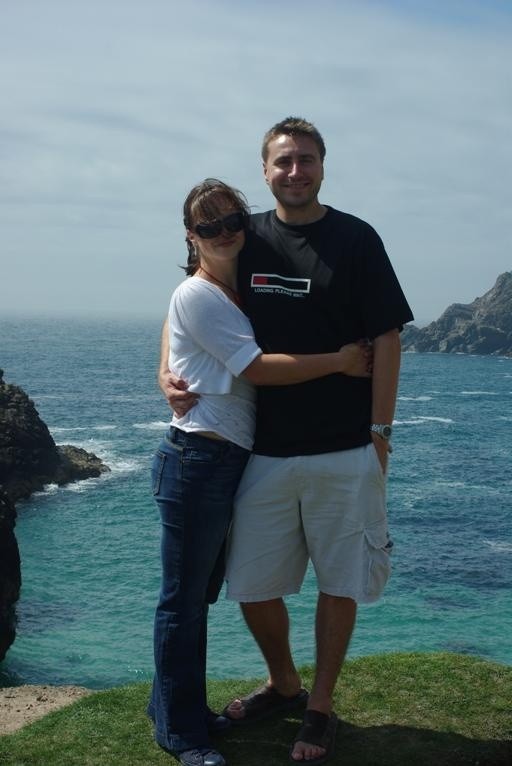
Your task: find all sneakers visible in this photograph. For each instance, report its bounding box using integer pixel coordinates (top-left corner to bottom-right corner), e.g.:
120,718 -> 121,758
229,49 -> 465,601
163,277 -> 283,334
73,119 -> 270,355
207,705 -> 230,728
175,747 -> 226,766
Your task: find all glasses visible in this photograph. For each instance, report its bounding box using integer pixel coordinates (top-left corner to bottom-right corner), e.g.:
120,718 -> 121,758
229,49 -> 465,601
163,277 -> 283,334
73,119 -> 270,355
196,212 -> 245,238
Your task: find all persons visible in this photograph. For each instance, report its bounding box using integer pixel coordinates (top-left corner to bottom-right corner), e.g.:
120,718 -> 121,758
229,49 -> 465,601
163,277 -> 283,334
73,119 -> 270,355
161,113 -> 413,766
145,179 -> 376,765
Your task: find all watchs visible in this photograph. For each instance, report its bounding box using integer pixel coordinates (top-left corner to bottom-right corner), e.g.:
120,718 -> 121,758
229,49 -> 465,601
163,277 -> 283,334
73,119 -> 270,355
368,421 -> 392,439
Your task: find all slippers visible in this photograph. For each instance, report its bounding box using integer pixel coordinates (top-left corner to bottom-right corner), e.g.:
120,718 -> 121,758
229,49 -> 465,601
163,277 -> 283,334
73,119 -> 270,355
288,707 -> 338,766
222,680 -> 307,724
